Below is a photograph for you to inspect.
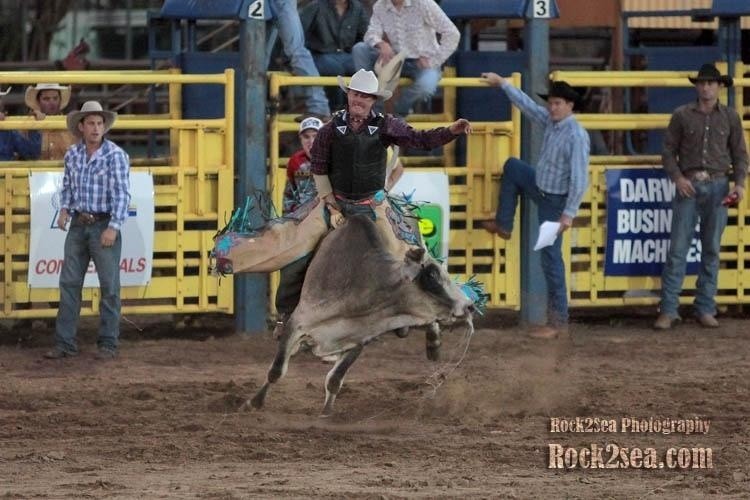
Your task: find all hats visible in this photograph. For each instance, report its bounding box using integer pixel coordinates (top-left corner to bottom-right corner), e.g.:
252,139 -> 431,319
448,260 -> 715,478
24,83 -> 72,111
536,81 -> 592,110
688,64 -> 733,87
66,100 -> 118,137
337,68 -> 393,103
374,50 -> 405,93
298,117 -> 324,134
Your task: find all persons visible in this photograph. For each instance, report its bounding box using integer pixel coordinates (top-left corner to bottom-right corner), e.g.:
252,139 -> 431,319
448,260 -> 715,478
20,83 -> 76,329
272,1 -> 472,341
43,100 -> 131,360
0,87 -> 45,337
654,62 -> 750,330
481,71 -> 592,334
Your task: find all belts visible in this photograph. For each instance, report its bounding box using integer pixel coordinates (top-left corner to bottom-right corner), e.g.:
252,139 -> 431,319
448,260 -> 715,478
686,170 -> 724,181
75,211 -> 110,224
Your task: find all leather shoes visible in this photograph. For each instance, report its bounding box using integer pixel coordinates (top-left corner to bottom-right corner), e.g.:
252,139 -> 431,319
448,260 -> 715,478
481,220 -> 511,241
94,350 -> 112,359
654,313 -> 672,330
294,112 -> 330,123
44,346 -> 64,359
272,319 -> 285,340
696,313 -> 719,328
526,323 -> 570,340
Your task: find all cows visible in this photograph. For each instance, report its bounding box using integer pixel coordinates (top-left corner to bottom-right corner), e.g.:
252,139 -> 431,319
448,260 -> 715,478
237,206 -> 479,421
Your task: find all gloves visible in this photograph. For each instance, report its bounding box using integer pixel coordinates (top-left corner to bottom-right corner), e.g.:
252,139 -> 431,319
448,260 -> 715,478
330,213 -> 345,229
